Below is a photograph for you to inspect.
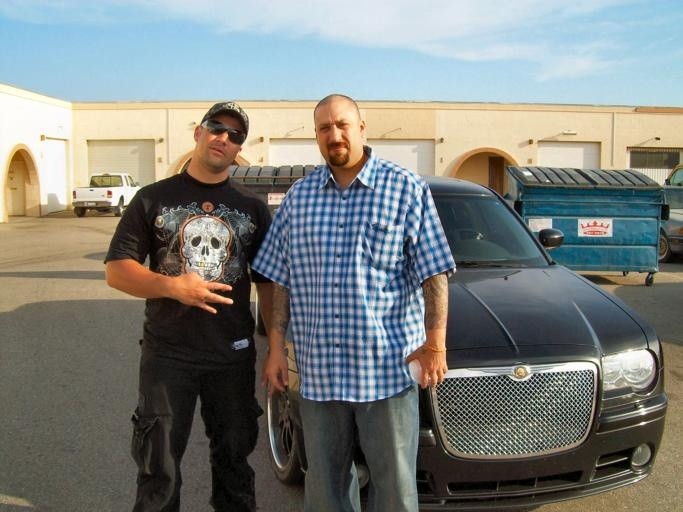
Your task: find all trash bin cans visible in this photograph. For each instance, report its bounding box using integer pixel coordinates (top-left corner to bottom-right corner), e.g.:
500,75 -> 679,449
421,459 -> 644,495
226,164 -> 321,268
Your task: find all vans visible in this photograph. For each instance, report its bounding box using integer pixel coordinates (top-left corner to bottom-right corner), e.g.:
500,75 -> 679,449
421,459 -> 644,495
659,164 -> 683,263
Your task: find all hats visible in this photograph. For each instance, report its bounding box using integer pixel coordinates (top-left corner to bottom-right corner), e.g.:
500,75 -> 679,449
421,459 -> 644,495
201,101 -> 249,135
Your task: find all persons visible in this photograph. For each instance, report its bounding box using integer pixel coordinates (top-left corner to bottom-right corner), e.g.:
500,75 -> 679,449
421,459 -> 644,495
102,102 -> 288,511
250,94 -> 457,512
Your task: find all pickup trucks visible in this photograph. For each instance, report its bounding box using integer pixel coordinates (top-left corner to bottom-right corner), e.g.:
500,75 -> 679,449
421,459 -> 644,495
72,173 -> 141,217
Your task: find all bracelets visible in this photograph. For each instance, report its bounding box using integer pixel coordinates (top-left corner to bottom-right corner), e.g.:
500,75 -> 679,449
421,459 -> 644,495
422,341 -> 447,353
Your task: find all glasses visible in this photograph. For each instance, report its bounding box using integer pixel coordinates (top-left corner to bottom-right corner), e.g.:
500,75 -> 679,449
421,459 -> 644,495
200,118 -> 246,145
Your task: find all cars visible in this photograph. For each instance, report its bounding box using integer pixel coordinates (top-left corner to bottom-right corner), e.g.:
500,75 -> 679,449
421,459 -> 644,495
254,176 -> 668,512
